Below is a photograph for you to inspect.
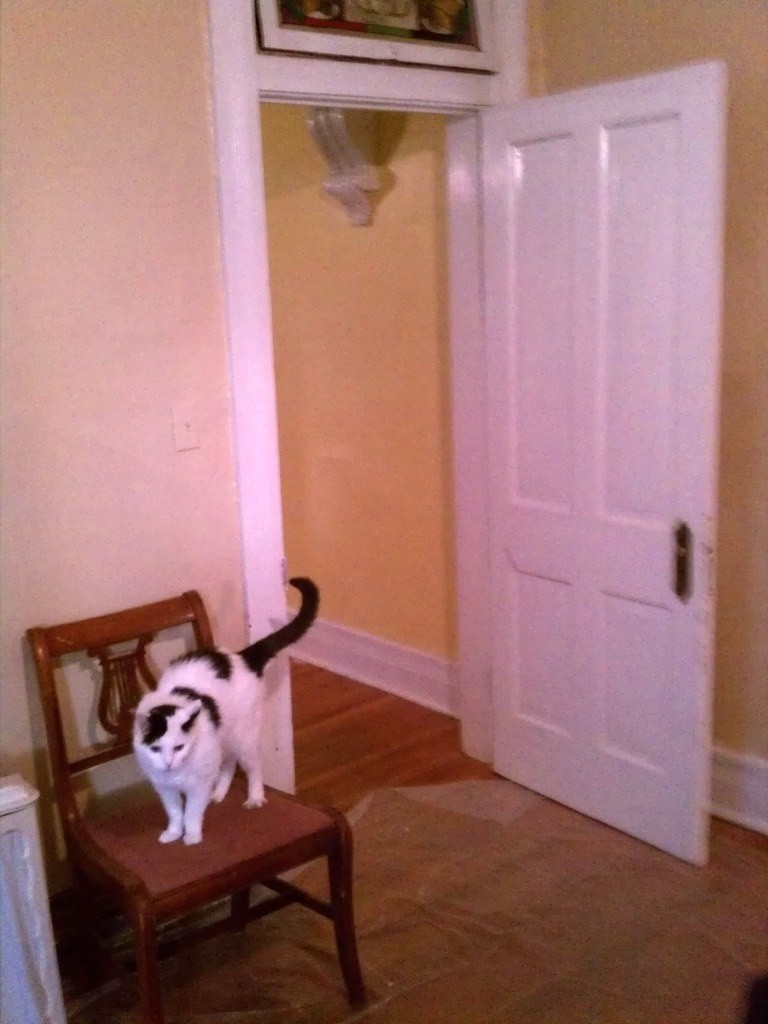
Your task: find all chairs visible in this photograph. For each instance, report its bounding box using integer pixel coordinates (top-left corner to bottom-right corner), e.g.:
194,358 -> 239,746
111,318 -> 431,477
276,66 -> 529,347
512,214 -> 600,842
27,587 -> 366,1024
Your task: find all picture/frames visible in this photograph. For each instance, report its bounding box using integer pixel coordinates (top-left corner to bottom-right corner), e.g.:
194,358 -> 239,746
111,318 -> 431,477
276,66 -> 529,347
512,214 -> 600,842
255,0 -> 502,75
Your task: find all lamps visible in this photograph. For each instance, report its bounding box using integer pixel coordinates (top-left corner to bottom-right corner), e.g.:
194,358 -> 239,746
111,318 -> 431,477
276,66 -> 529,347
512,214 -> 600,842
303,105 -> 399,225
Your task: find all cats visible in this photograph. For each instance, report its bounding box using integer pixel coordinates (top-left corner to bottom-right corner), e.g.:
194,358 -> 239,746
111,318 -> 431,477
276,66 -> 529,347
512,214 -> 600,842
130,576 -> 320,847
